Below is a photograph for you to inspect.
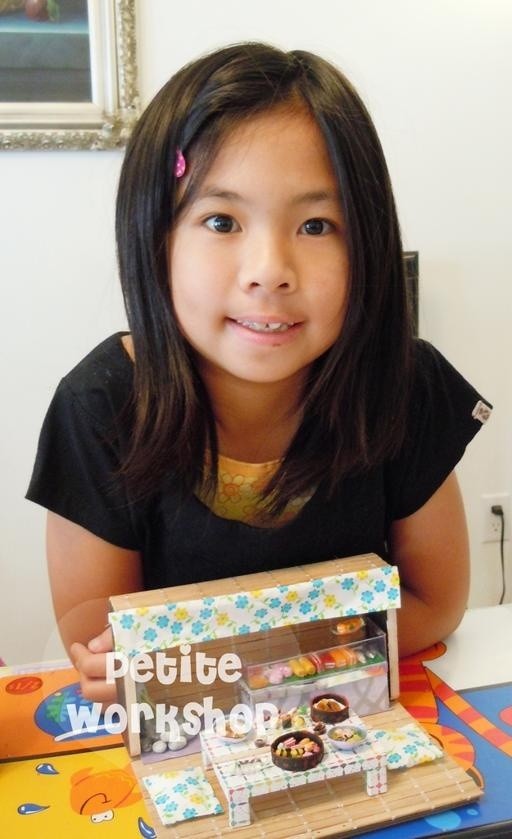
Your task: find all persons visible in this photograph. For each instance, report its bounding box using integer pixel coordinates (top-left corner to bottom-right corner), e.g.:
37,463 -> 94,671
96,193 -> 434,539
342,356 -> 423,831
24,42 -> 495,708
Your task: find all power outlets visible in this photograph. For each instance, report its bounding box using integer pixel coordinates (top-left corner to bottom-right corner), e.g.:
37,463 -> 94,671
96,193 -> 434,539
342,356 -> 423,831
480,495 -> 512,543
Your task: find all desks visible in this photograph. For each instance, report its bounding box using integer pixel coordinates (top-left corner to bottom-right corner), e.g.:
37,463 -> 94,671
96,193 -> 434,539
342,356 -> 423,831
0,601 -> 512,839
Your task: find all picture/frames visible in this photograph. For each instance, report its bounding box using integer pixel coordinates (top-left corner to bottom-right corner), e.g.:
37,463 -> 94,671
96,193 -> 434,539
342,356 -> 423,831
0,0 -> 141,150
